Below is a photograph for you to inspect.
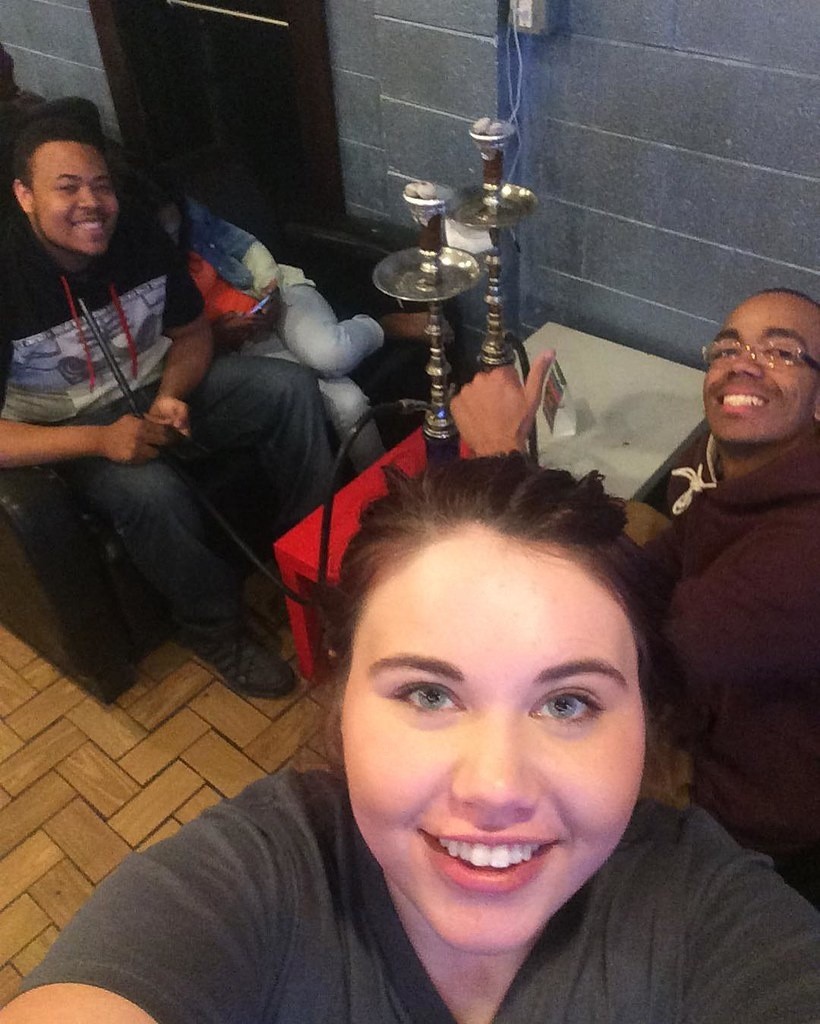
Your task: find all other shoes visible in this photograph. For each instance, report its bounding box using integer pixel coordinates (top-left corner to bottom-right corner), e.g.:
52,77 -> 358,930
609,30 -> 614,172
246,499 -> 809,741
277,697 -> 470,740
196,630 -> 296,696
424,313 -> 455,344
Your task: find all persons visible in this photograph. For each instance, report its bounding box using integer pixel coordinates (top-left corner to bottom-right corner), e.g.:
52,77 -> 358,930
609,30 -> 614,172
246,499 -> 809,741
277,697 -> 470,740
449,289 -> 820,913
0,115 -> 454,701
0,449 -> 820,1023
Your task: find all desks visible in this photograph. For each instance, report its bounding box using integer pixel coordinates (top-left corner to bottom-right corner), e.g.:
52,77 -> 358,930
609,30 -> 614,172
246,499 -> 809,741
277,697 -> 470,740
274,320 -> 708,684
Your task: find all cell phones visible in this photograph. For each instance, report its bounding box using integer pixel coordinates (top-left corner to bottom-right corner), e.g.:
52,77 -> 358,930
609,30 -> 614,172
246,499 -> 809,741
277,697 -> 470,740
247,288 -> 280,317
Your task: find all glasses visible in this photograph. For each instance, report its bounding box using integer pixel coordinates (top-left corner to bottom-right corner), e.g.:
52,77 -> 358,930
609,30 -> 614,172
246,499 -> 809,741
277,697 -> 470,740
700,335 -> 820,372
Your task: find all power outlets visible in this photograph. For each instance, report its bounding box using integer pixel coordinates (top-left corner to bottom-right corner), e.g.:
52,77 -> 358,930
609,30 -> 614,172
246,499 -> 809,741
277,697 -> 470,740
518,0 -> 533,28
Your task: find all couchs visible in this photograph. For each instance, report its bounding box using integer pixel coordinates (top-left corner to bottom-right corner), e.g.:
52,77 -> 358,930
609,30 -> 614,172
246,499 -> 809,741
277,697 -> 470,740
0,201 -> 413,704
